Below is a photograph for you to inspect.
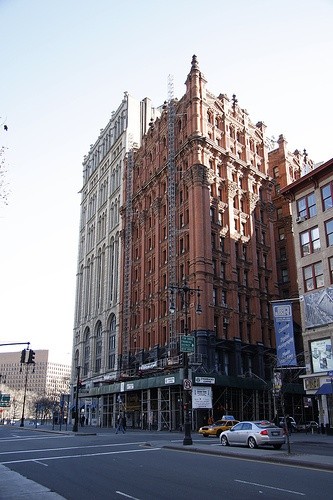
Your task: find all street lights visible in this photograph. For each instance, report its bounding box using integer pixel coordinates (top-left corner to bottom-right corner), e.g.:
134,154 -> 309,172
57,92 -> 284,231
166,278 -> 204,446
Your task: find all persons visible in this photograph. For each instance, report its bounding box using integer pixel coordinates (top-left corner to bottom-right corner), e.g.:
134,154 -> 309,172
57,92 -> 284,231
286,415 -> 293,431
81,415 -> 85,427
115,417 -> 126,434
208,415 -> 214,425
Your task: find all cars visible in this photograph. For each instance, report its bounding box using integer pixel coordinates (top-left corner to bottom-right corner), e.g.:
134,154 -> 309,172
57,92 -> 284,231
270,416 -> 296,432
218,418 -> 287,450
198,414 -> 242,437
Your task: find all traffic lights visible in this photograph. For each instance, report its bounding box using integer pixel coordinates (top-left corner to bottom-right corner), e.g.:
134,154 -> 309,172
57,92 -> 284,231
137,370 -> 145,380
28,349 -> 35,363
